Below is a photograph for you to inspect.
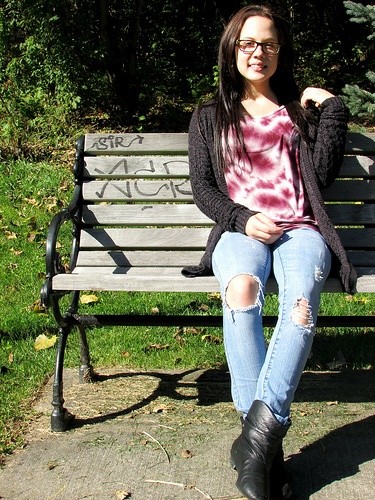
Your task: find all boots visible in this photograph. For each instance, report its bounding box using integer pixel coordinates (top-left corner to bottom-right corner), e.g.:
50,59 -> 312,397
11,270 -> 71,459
240,417 -> 292,500
230,400 -> 292,500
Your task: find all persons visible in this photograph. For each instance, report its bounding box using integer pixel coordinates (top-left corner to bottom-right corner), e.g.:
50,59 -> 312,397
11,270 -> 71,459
182,5 -> 356,499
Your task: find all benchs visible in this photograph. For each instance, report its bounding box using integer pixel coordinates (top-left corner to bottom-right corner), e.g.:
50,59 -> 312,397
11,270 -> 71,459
40,133 -> 375,432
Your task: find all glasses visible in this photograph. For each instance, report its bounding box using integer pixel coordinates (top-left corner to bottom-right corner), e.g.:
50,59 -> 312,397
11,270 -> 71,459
235,40 -> 281,55
